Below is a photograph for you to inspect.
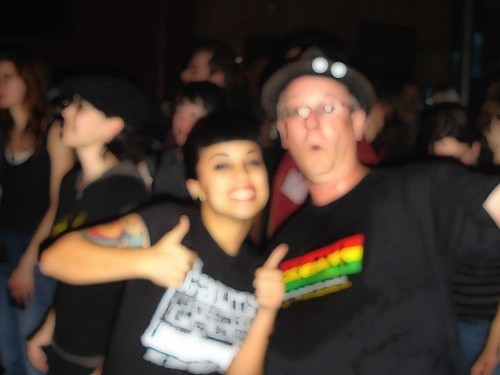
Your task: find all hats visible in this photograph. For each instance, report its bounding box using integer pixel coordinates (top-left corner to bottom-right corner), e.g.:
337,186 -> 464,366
60,73 -> 146,131
262,35 -> 375,122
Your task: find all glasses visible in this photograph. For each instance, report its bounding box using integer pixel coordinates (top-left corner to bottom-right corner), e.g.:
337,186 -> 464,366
277,100 -> 355,119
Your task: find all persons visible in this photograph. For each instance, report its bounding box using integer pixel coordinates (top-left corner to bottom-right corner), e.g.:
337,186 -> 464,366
38,112 -> 295,375
28,18 -> 500,244
0,50 -> 84,375
254,50 -> 500,375
23,63 -> 151,375
